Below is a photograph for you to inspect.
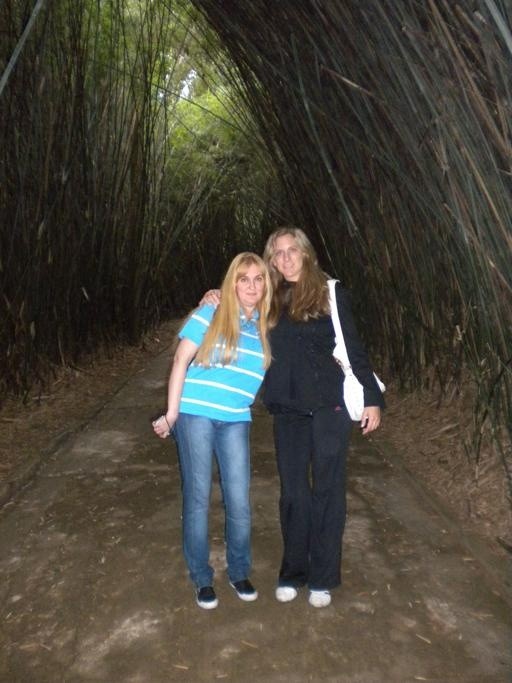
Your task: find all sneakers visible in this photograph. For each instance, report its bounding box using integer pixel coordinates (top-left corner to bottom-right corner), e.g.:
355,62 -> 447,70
309,588 -> 333,609
228,578 -> 259,602
193,587 -> 218,612
276,585 -> 298,604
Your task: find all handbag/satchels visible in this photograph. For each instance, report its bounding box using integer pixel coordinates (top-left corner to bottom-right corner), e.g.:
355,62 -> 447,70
331,337 -> 386,424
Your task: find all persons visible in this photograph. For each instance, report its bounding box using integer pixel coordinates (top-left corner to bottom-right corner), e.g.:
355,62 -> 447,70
193,223 -> 387,608
148,248 -> 273,610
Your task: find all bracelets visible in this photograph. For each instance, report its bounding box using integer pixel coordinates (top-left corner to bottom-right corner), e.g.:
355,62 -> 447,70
163,415 -> 173,434
